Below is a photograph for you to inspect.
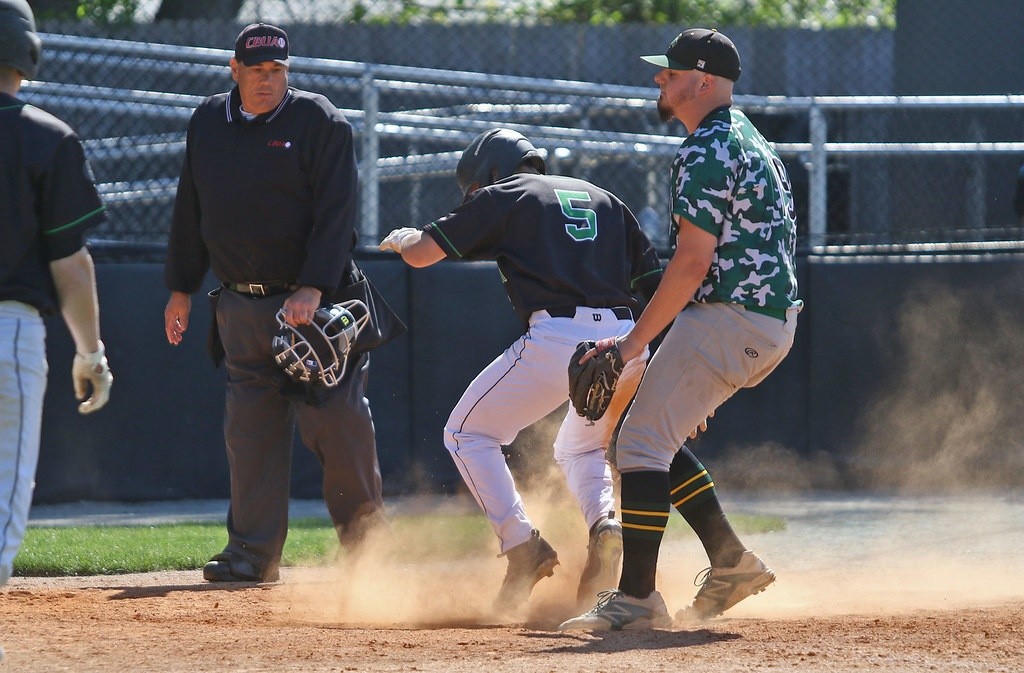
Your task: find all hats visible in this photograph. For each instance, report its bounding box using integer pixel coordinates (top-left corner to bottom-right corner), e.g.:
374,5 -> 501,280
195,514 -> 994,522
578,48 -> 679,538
235,23 -> 289,67
640,29 -> 742,82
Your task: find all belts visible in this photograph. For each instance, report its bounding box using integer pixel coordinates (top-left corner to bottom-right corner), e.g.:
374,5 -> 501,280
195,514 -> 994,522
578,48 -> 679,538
546,305 -> 632,320
746,305 -> 786,321
225,282 -> 296,298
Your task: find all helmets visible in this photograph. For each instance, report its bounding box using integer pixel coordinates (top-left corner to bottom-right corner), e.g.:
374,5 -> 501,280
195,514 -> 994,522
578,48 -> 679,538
0,0 -> 42,81
457,128 -> 546,198
271,309 -> 360,399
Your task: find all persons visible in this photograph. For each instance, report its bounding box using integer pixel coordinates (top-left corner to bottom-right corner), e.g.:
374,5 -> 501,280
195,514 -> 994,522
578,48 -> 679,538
163,23 -> 409,582
0,0 -> 114,661
380,29 -> 804,631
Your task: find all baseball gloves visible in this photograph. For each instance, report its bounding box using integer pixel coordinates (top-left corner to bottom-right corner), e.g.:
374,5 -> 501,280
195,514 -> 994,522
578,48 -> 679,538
567,341 -> 626,421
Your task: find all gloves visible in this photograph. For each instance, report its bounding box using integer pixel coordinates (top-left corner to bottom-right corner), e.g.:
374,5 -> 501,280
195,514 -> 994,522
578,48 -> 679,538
72,340 -> 114,414
380,228 -> 418,253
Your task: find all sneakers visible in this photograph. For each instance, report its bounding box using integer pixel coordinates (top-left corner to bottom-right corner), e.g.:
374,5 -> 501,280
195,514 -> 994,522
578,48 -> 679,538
675,549 -> 777,624
559,589 -> 672,629
577,512 -> 622,612
203,560 -> 242,581
492,530 -> 559,609
